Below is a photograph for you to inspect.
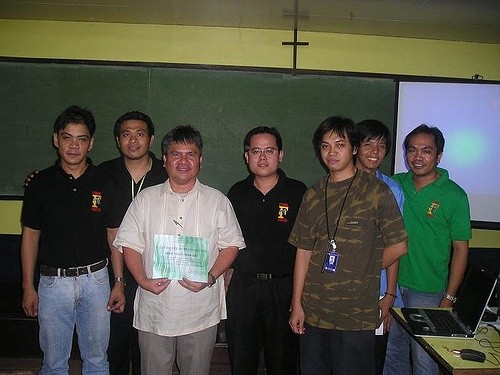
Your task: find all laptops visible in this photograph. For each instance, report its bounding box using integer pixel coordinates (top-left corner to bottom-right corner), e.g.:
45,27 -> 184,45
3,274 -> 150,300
401,259 -> 498,340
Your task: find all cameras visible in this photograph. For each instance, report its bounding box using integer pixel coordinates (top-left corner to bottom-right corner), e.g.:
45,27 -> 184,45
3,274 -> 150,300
323,251 -> 340,272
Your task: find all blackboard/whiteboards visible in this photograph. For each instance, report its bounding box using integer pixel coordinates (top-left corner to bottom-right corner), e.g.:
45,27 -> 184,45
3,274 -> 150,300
0,58 -> 396,201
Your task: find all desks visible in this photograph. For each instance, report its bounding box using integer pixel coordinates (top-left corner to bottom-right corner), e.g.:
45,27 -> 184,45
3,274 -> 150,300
389,307 -> 500,375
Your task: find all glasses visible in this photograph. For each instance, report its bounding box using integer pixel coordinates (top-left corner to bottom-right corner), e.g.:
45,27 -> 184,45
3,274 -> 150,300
250,149 -> 279,155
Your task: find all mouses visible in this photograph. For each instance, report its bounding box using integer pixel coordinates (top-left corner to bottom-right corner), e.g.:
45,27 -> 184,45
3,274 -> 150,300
460,349 -> 486,363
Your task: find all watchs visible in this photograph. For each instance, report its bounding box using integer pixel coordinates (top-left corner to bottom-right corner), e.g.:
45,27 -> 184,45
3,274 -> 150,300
442,292 -> 458,304
112,276 -> 129,285
208,271 -> 218,288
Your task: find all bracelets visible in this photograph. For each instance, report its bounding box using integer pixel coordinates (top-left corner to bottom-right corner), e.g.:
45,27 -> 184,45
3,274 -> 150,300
384,290 -> 398,300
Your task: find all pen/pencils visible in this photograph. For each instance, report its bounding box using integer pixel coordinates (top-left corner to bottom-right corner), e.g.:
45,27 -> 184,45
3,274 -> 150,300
451,334 -> 474,337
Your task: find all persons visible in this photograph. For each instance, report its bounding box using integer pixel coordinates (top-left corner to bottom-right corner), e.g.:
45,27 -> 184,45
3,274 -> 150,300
19,103 -> 127,374
357,117 -> 406,334
225,125 -> 307,374
388,122 -> 473,374
286,113 -> 408,374
99,109 -> 167,375
111,123 -> 246,375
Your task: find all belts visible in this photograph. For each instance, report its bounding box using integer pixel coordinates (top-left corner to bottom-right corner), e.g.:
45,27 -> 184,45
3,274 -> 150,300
233,268 -> 294,280
39,258 -> 107,276
